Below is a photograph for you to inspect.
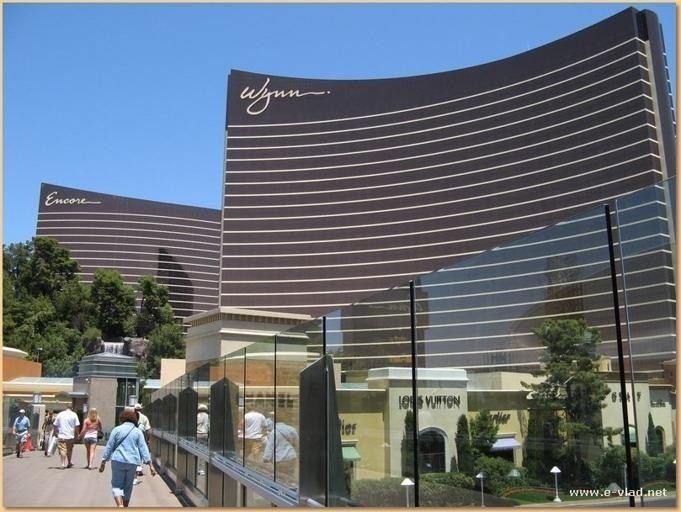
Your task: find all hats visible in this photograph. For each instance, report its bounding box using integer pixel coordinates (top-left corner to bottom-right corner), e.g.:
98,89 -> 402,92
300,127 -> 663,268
268,411 -> 275,415
18,409 -> 25,414
197,404 -> 209,412
133,403 -> 143,410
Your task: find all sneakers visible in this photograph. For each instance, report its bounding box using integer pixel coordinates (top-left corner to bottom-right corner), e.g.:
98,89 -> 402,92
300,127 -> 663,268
198,471 -> 206,477
62,463 -> 74,468
133,471 -> 144,485
87,465 -> 93,469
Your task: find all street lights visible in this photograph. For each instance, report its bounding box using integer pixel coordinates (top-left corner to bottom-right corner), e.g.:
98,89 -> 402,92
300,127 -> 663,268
622,463 -> 627,488
476,472 -> 488,508
400,478 -> 415,507
549,466 -> 562,503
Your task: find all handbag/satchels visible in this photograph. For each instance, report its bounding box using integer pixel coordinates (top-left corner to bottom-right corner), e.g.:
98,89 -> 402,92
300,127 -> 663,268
97,428 -> 104,439
25,434 -> 33,452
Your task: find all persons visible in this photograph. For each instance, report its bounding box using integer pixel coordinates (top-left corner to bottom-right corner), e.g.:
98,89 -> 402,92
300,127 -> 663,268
42,408 -> 56,457
76,408 -> 102,469
13,409 -> 31,457
262,412 -> 299,507
196,403 -> 209,476
239,404 -> 267,464
131,412 -> 145,485
238,406 -> 245,458
52,403 -> 81,468
98,410 -> 156,507
133,402 -> 152,477
